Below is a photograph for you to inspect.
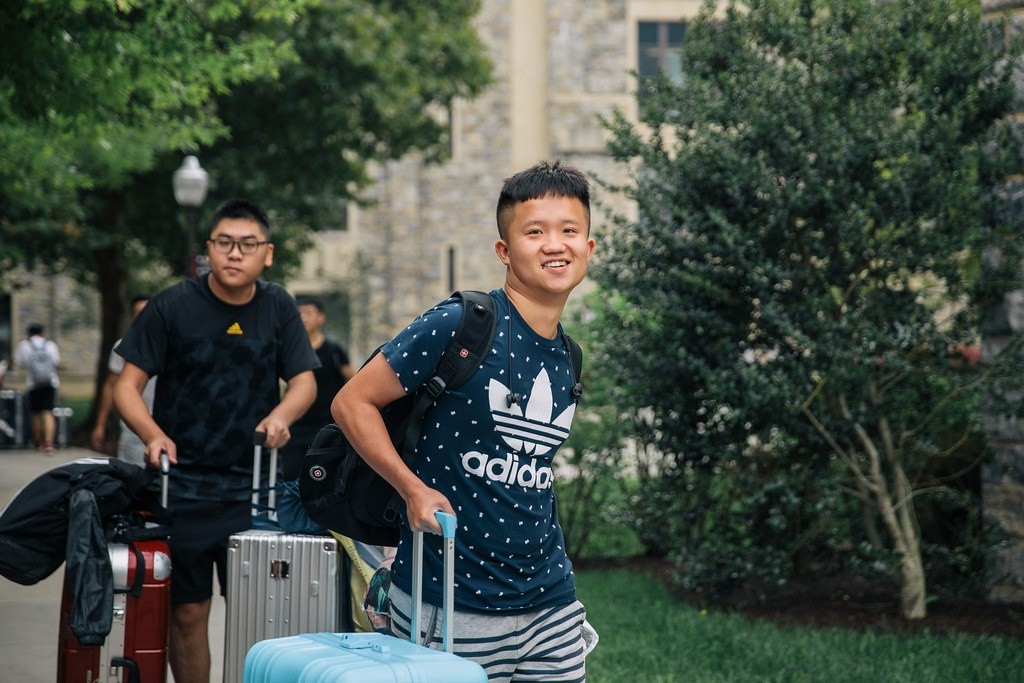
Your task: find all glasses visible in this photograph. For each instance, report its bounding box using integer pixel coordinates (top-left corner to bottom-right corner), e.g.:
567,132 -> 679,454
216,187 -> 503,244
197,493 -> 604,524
207,237 -> 273,254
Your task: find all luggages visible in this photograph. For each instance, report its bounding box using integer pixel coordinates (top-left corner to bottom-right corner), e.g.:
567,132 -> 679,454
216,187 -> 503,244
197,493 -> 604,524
56,450 -> 175,683
222,432 -> 352,683
242,510 -> 488,683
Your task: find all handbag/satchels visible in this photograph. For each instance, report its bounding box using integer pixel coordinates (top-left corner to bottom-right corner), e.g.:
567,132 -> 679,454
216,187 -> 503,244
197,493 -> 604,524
247,477 -> 332,536
364,548 -> 398,639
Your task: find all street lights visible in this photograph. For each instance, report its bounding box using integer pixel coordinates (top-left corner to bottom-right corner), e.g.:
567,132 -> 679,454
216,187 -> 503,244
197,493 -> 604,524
171,154 -> 209,277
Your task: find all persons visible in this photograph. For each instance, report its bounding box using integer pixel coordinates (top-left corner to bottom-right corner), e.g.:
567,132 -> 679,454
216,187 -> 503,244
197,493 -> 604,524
329,156 -> 599,683
12,320 -> 62,453
90,295 -> 158,469
277,292 -> 353,484
112,197 -> 324,683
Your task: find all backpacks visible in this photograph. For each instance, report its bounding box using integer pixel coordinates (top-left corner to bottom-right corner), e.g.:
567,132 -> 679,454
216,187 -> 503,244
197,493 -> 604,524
0,457 -> 176,597
27,337 -> 51,384
298,289 -> 583,547
